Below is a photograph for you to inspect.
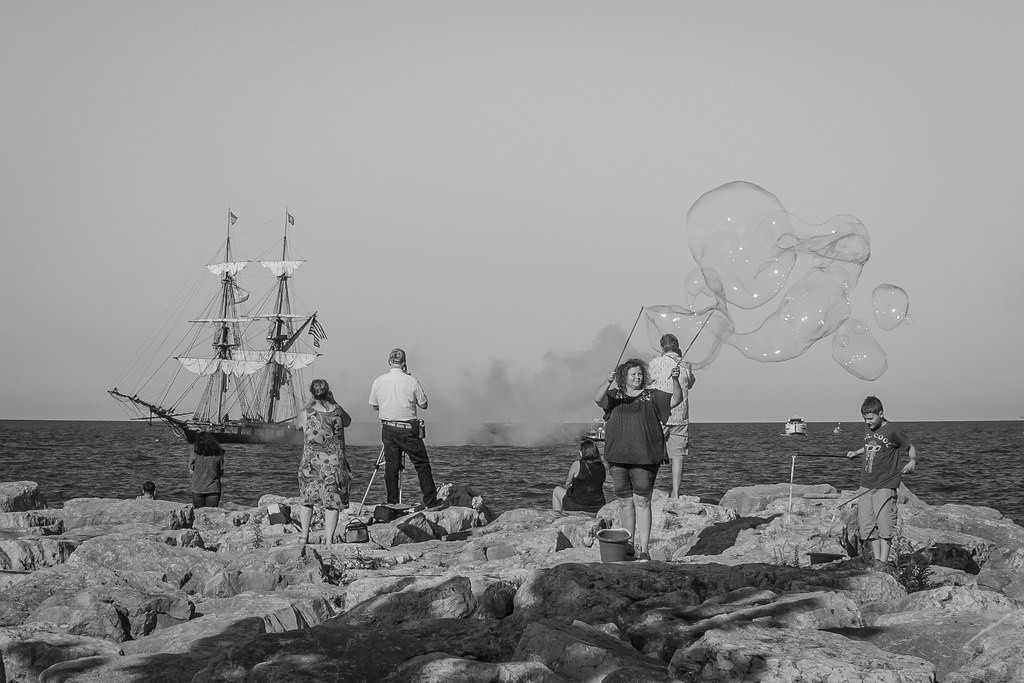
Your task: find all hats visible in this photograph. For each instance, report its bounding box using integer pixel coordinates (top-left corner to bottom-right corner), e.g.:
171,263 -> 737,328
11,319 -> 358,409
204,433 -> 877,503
389,348 -> 406,364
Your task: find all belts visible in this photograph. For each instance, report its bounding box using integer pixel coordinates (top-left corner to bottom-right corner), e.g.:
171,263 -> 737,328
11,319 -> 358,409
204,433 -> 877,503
382,422 -> 412,429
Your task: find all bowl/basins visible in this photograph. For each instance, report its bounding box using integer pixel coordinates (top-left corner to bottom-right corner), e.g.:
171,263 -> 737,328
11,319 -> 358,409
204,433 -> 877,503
806,552 -> 846,565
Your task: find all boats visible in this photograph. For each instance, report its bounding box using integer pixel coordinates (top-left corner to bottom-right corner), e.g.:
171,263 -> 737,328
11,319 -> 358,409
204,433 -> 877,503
833,423 -> 843,434
785,416 -> 807,435
585,417 -> 607,442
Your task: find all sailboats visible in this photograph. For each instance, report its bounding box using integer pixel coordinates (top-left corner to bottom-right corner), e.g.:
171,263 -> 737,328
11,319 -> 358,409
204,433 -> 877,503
106,204 -> 325,447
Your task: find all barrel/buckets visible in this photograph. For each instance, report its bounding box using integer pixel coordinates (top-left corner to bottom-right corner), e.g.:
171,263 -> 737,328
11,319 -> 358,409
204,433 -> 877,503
596,528 -> 632,563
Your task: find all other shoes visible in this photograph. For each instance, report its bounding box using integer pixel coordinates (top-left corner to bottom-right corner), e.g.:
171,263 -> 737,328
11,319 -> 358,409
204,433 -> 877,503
626,544 -> 635,556
298,537 -> 309,545
639,552 -> 651,562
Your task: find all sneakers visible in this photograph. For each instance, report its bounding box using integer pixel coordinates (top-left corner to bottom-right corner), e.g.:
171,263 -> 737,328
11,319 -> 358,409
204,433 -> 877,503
427,498 -> 444,510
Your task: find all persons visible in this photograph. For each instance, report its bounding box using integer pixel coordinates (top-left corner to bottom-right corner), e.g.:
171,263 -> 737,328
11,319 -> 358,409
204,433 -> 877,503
136,482 -> 156,500
552,440 -> 607,513
594,358 -> 684,563
645,334 -> 696,500
369,349 -> 444,512
187,432 -> 226,508
296,380 -> 352,544
847,396 -> 917,572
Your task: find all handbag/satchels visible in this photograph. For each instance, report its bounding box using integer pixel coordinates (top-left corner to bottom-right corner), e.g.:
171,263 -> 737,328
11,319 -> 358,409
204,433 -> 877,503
374,506 -> 397,520
345,518 -> 369,543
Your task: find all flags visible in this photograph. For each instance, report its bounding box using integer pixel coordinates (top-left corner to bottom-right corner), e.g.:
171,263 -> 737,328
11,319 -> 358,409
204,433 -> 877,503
287,214 -> 294,225
228,211 -> 238,225
308,318 -> 328,348
